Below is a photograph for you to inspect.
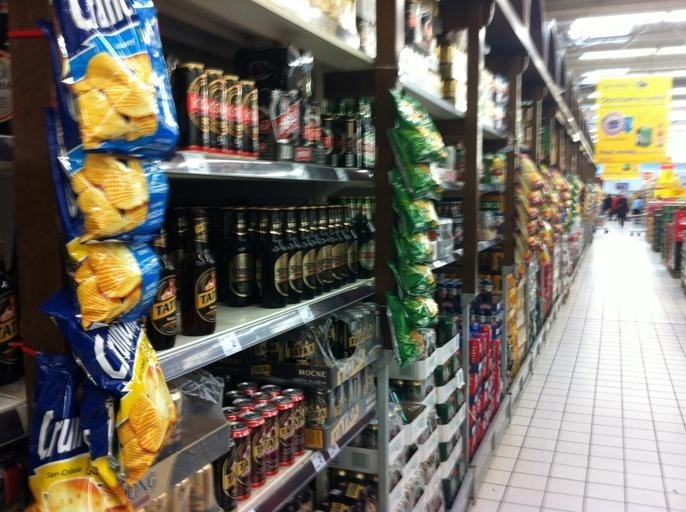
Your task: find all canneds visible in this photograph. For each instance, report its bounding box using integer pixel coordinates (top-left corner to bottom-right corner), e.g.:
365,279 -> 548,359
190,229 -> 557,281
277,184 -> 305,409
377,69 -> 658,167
176,63 -> 259,159
214,301 -> 384,512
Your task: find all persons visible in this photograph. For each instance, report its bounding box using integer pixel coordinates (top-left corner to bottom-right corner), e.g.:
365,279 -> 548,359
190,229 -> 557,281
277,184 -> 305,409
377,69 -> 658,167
601,194 -> 644,227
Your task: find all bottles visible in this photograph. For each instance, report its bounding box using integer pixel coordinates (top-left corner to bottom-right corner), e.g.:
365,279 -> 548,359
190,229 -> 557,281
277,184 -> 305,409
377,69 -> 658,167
146,192 -> 378,354
0,231 -> 22,389
437,200 -> 499,247
335,96 -> 377,168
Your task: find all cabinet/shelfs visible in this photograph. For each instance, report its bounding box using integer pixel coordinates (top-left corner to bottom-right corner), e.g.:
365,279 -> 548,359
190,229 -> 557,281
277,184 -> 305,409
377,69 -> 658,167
518,0 -> 606,408
387,1 -> 477,510
473,1 -> 518,497
2,5 -> 387,512
